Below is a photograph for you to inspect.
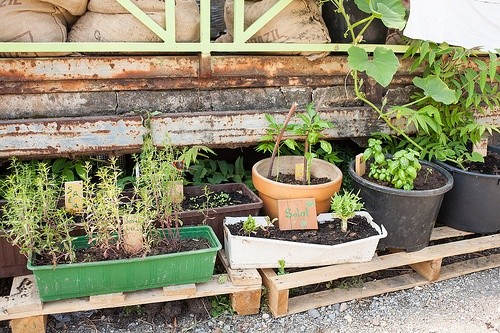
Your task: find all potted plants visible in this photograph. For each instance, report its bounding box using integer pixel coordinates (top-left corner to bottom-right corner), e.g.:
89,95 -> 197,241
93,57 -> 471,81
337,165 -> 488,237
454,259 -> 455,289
223,188 -> 388,270
319,0 -> 500,234
253,102 -> 343,224
0,130 -> 222,302
348,132 -> 453,253
0,183 -> 263,278
